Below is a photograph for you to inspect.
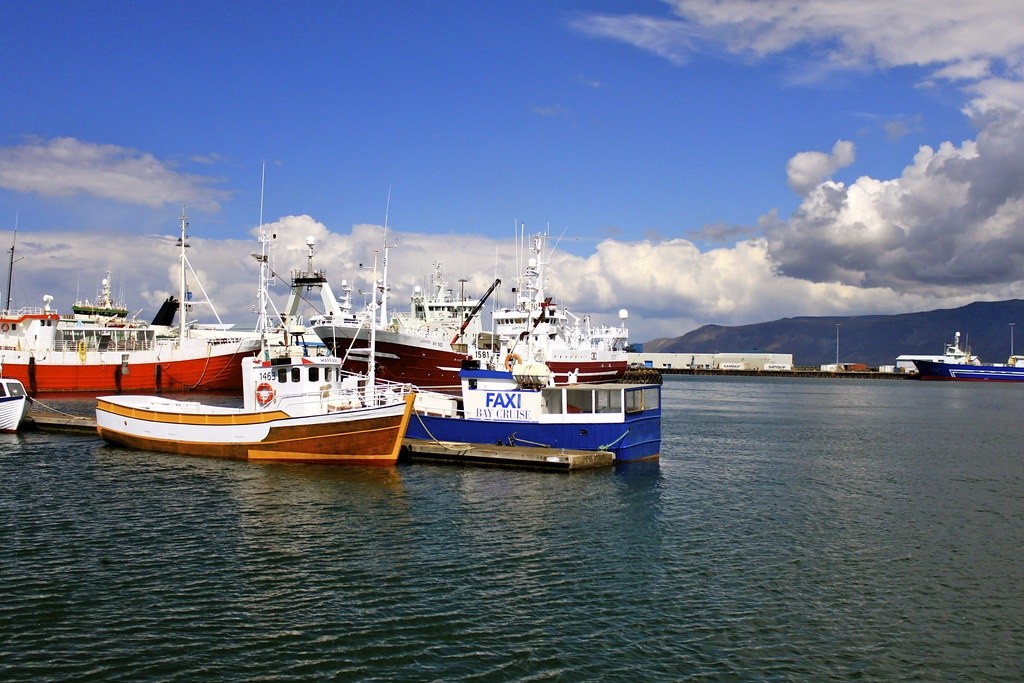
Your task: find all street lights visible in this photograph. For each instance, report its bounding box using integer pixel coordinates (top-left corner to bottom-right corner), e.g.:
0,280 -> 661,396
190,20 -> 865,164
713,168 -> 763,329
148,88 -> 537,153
1008,322 -> 1016,356
834,323 -> 841,365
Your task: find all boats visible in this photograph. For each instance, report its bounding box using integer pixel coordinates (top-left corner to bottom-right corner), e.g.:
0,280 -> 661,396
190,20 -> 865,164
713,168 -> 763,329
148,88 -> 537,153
0,160 -> 665,473
911,327 -> 1024,382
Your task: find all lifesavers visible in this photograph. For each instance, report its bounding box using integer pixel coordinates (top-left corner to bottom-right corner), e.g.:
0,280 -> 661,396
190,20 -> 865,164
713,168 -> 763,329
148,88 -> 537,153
255,383 -> 273,404
504,353 -> 521,372
1,323 -> 10,332
